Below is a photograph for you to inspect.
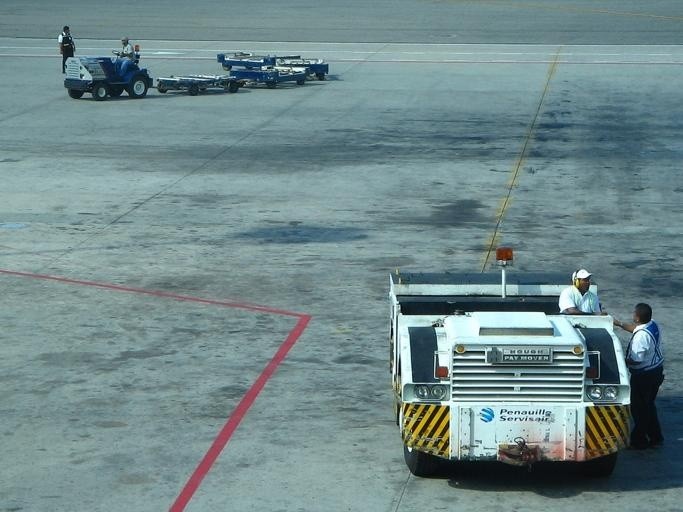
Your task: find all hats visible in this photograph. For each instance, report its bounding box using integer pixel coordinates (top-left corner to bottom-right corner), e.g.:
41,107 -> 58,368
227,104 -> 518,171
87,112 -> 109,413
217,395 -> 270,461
571,268 -> 592,281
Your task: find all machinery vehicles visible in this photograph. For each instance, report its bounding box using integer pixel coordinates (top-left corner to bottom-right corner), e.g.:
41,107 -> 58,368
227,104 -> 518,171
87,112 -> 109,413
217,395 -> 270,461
63,51 -> 153,99
387,264 -> 636,482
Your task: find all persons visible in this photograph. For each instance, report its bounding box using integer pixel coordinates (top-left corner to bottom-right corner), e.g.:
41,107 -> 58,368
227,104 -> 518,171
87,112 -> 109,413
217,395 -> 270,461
612,302 -> 664,450
114,37 -> 136,82
57,26 -> 76,73
559,268 -> 607,315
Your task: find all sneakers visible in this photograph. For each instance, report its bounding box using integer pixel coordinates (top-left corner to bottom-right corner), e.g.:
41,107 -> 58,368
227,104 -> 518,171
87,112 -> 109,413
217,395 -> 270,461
623,443 -> 648,452
648,440 -> 664,448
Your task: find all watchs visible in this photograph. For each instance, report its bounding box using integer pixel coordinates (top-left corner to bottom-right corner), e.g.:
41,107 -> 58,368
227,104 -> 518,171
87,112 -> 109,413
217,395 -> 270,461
619,321 -> 624,329
